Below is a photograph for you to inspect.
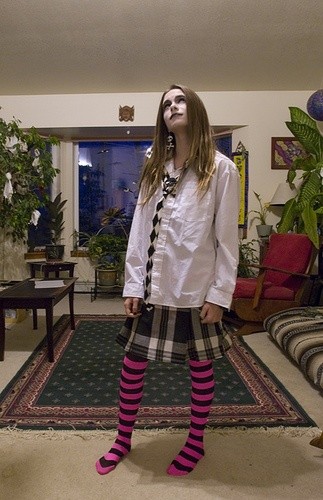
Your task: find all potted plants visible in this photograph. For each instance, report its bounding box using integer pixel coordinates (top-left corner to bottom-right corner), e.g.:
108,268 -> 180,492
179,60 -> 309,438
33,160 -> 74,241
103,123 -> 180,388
45,192 -> 68,259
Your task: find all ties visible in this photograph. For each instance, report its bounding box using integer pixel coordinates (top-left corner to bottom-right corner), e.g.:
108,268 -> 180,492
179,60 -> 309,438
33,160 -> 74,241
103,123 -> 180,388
142,157 -> 192,311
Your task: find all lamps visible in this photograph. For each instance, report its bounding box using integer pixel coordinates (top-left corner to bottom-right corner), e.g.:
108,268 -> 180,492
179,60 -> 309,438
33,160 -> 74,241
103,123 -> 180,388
270,182 -> 300,233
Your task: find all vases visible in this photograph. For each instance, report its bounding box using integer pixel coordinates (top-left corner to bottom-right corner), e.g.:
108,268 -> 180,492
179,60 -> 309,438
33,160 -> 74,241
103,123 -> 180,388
119,271 -> 125,286
96,268 -> 118,286
256,225 -> 273,236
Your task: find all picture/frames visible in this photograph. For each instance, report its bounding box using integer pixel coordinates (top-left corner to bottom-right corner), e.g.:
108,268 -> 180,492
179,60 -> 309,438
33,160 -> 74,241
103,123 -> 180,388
271,137 -> 310,169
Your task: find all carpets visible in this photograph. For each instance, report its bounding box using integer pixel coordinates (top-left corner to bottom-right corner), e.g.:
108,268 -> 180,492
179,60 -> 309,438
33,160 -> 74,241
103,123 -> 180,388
0,314 -> 323,440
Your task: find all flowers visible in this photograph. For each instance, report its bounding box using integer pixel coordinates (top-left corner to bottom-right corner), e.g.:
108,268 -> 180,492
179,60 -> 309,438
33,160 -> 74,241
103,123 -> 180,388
68,207 -> 129,273
247,191 -> 271,230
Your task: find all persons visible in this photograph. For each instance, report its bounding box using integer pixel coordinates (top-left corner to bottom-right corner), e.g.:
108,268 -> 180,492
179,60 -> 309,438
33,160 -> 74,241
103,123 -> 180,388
96,85 -> 241,476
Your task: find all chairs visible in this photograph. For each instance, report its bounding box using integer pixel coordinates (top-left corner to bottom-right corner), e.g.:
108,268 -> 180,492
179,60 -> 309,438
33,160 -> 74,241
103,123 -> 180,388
229,233 -> 319,336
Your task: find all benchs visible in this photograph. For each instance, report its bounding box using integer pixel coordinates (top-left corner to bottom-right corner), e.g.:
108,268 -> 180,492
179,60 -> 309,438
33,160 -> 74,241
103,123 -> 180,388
263,306 -> 323,399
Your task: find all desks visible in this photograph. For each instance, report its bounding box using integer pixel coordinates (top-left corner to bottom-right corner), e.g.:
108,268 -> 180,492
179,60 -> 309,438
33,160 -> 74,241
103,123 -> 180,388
0,278 -> 79,362
31,260 -> 78,278
